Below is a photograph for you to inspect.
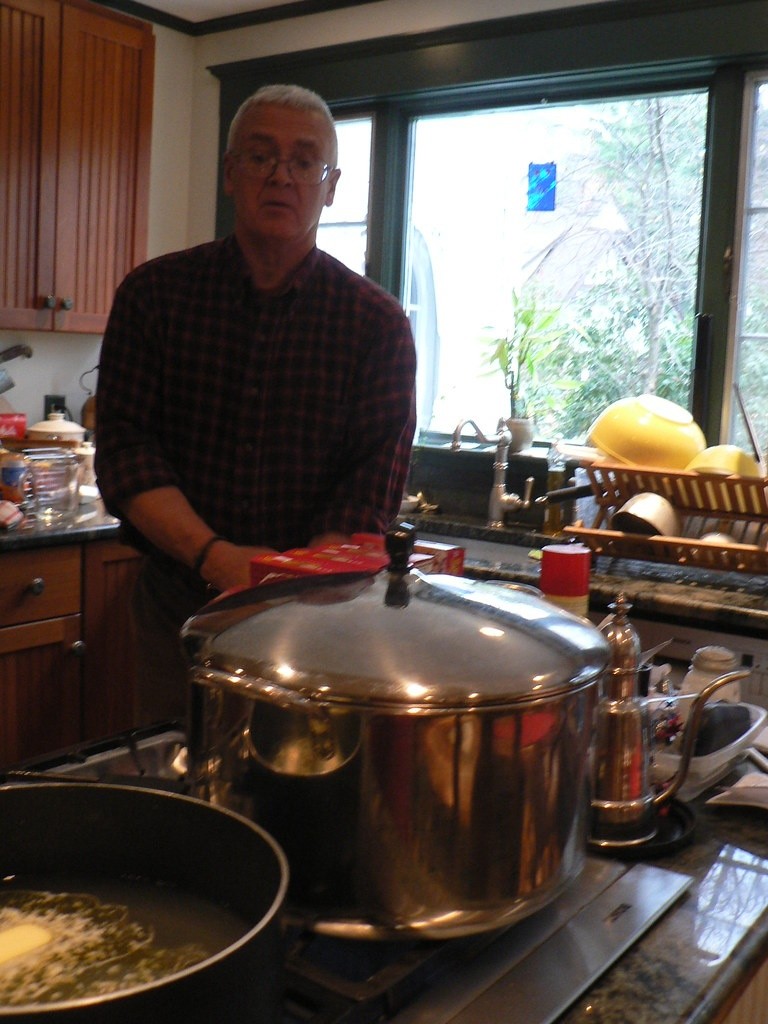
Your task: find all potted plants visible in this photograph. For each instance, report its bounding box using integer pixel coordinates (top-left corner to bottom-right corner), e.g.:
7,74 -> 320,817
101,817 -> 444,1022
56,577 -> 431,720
484,292 -> 560,451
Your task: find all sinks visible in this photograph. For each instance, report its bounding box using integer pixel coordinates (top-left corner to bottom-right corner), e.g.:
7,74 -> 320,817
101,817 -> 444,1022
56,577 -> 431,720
396,517 -> 570,567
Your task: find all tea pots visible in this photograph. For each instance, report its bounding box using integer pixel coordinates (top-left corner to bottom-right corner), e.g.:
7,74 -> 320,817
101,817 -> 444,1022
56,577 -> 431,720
590,595 -> 752,829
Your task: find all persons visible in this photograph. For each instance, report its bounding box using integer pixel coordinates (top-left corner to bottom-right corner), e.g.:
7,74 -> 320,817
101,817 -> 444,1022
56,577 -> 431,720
93,85 -> 417,722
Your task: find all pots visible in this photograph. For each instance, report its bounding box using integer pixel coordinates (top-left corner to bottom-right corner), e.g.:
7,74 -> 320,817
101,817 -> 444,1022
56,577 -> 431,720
174,515 -> 608,955
1,773 -> 289,1024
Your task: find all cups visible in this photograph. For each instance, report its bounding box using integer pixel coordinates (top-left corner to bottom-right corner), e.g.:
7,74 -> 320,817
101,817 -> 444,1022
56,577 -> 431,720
24,413 -> 85,454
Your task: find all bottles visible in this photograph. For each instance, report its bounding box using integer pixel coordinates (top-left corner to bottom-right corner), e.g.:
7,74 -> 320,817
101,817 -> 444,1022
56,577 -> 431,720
24,448 -> 81,523
681,643 -> 742,706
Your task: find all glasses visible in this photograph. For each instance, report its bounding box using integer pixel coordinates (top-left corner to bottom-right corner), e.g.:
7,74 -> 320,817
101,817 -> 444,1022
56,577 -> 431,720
229,148 -> 338,185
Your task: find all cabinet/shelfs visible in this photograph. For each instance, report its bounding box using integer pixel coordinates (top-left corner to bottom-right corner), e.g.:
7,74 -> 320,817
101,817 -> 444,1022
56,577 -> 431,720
0,0 -> 156,332
0,541 -> 197,766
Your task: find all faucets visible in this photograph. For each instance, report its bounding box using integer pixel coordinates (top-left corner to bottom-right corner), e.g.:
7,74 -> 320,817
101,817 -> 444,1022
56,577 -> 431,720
450,416 -> 534,530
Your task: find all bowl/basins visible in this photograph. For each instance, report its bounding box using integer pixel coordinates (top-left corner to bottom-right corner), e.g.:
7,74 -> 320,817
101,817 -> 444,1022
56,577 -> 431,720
585,391 -> 763,561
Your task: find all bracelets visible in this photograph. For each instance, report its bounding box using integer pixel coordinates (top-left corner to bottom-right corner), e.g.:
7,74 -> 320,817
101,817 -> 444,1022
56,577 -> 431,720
193,536 -> 223,587
